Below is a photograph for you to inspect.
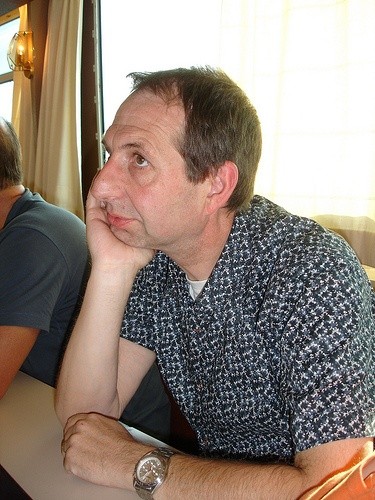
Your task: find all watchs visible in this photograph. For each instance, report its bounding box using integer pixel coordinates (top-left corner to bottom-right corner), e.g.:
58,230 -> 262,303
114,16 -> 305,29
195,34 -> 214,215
133,445 -> 177,500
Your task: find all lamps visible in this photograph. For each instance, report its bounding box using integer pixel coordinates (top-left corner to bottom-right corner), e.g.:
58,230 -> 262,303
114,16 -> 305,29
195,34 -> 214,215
6,31 -> 37,80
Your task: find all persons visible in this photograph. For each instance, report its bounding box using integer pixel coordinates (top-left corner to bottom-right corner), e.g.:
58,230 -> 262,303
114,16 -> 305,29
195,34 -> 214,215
54,63 -> 375,500
0,116 -> 92,400
